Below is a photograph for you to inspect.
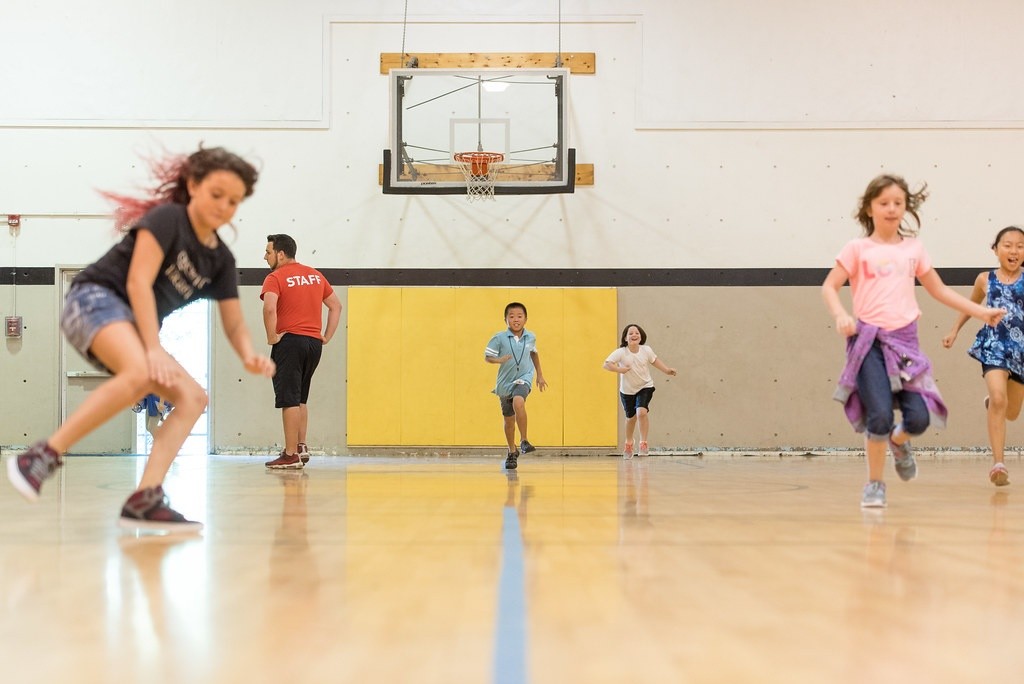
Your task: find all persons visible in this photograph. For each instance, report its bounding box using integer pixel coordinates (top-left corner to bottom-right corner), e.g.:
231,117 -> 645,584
483,302 -> 548,469
260,233 -> 344,472
601,323 -> 677,460
818,170 -> 1015,507
6,144 -> 274,535
939,224 -> 1024,487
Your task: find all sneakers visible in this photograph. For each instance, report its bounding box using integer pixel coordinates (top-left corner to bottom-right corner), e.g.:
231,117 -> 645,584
638,442 -> 649,456
990,467 -> 1010,486
520,439 -> 535,453
8,441 -> 63,505
265,452 -> 303,469
505,445 -> 519,469
118,485 -> 204,533
862,479 -> 887,508
887,423 -> 917,482
280,443 -> 310,466
623,439 -> 635,460
